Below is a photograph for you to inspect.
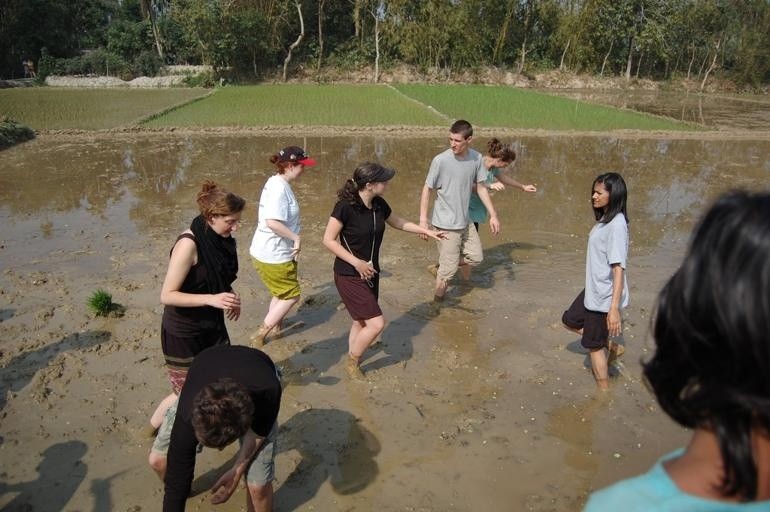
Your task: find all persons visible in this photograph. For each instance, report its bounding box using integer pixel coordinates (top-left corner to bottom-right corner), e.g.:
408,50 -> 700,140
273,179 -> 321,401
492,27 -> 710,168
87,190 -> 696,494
582,179 -> 769,512
468,135 -> 538,233
150,345 -> 281,511
147,178 -> 248,437
248,146 -> 319,346
562,172 -> 630,388
323,162 -> 452,385
418,119 -> 502,305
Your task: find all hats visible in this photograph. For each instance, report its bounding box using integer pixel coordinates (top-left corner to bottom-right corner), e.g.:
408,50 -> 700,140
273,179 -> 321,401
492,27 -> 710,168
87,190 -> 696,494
278,146 -> 318,166
357,166 -> 395,184
